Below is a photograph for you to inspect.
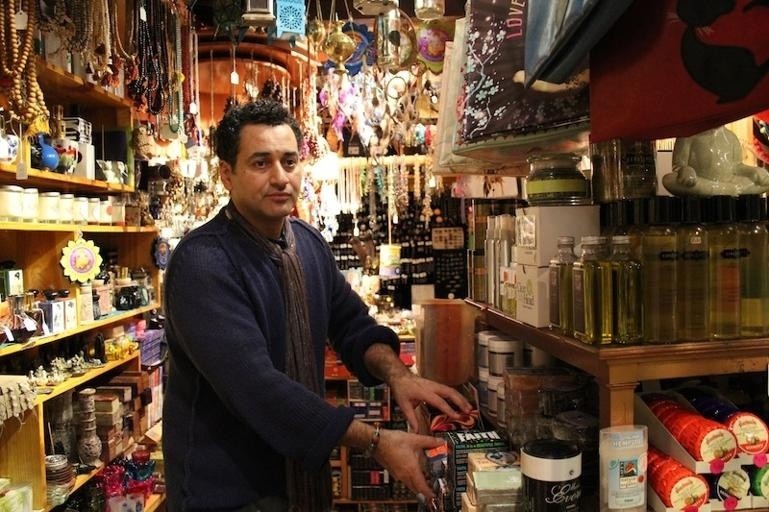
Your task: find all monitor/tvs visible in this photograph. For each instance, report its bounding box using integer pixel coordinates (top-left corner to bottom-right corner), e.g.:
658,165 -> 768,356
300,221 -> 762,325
246,0 -> 270,13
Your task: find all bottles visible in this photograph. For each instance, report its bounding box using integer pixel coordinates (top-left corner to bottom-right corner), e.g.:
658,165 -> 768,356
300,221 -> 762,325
547,234 -> 578,334
526,152 -> 591,206
519,438 -> 584,512
608,234 -> 643,346
0,185 -> 127,226
642,222 -> 769,345
570,235 -> 613,347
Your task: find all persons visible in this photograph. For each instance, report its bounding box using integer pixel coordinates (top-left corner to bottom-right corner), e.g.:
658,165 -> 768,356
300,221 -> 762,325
161,97 -> 473,511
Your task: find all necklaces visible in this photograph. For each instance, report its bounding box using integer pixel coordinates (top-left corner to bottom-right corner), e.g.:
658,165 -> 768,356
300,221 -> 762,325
0,0 -> 200,139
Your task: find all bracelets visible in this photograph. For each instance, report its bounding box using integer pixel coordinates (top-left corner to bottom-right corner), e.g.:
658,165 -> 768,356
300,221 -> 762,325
362,420 -> 383,460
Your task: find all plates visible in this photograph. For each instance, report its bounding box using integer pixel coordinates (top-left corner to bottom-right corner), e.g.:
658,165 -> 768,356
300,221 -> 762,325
46,454 -> 74,484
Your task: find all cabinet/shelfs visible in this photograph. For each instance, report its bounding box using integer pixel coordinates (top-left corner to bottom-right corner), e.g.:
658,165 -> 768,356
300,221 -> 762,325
461,297 -> 769,512
0,54 -> 166,512
